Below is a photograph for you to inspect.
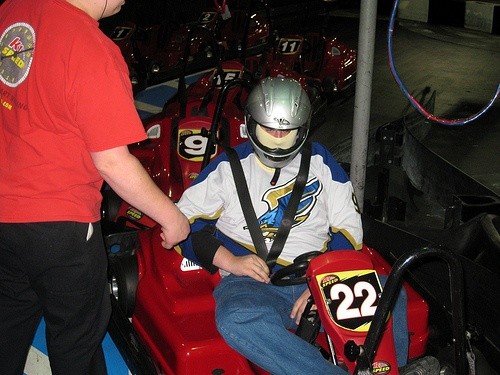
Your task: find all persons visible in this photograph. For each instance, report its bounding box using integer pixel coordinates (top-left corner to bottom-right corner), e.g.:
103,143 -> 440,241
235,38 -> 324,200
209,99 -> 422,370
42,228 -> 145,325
170,77 -> 409,375
0,0 -> 190,375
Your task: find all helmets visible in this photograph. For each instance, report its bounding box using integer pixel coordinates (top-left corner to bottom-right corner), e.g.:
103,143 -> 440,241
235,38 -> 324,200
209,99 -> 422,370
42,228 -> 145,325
244,77 -> 312,168
